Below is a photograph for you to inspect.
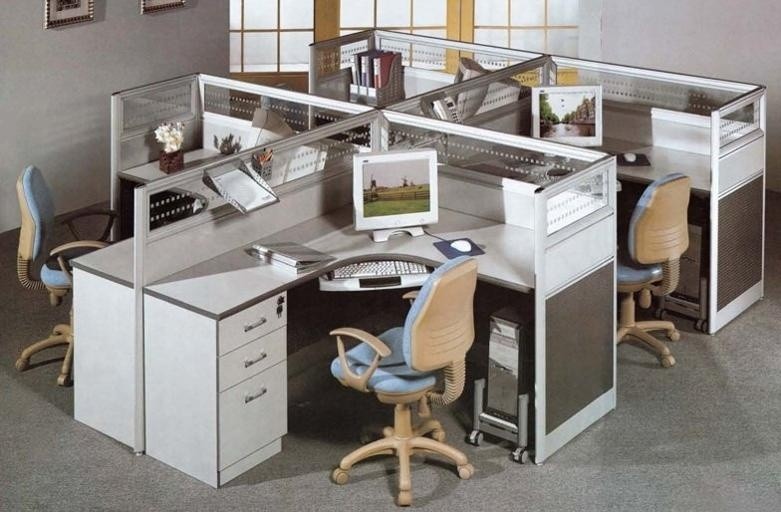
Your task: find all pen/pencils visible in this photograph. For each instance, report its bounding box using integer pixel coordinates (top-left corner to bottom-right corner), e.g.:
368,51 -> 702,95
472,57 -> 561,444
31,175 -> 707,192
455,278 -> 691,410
260,148 -> 276,161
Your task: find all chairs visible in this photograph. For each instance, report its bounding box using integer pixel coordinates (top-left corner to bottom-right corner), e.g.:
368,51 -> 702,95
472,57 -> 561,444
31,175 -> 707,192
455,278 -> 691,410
615,171 -> 691,367
16,165 -> 118,386
261,84 -> 307,132
331,256 -> 479,506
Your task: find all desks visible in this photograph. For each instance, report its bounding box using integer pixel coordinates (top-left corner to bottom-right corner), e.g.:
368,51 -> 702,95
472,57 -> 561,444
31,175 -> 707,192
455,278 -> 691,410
386,55 -> 766,336
143,108 -> 617,491
73,74 -> 377,450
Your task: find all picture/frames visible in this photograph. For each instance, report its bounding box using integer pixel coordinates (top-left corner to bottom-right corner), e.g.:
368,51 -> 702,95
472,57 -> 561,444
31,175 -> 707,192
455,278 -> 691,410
44,1 -> 95,29
140,0 -> 185,14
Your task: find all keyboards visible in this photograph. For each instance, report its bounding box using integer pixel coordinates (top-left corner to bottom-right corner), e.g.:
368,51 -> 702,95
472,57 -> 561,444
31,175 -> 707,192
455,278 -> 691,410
330,259 -> 428,279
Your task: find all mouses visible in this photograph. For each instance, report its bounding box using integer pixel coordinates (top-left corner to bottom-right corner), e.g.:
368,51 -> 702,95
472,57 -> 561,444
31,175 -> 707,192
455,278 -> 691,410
449,239 -> 472,253
624,152 -> 636,162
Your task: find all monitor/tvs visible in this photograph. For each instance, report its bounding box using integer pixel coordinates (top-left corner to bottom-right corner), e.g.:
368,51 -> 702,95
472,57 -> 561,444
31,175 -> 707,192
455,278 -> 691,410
531,84 -> 603,148
243,108 -> 327,185
448,56 -> 521,123
353,147 -> 437,241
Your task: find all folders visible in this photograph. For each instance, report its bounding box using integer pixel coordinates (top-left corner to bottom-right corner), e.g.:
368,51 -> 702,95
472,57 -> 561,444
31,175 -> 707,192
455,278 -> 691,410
375,54 -> 392,89
363,51 -> 391,87
250,240 -> 339,273
349,49 -> 376,85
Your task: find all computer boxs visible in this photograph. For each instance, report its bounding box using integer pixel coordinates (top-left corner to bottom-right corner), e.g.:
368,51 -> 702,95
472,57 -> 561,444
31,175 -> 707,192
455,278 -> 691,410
675,224 -> 703,299
487,300 -> 534,419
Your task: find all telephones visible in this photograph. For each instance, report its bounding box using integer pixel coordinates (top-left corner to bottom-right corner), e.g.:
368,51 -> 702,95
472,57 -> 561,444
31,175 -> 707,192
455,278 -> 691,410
433,97 -> 468,124
